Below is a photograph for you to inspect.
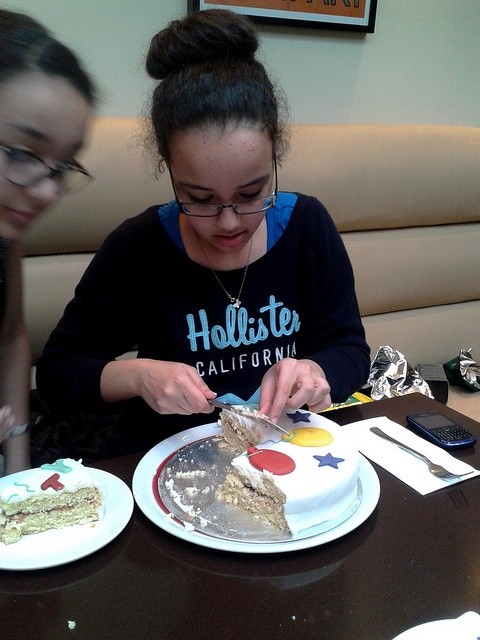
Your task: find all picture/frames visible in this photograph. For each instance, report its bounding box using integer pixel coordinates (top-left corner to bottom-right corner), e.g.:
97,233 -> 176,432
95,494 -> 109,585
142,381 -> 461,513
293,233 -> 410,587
187,0 -> 377,33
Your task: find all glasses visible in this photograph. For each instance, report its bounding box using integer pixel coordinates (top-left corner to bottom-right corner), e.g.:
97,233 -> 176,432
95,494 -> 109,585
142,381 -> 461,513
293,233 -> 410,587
1,143 -> 95,197
164,153 -> 282,217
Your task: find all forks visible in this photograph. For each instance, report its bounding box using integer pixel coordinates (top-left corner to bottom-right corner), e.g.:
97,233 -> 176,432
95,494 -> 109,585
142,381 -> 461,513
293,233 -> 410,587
370,426 -> 474,480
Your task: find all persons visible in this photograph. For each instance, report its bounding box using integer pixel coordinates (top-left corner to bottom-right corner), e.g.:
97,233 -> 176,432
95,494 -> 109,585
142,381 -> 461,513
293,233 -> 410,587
37,9 -> 373,457
0,8 -> 103,480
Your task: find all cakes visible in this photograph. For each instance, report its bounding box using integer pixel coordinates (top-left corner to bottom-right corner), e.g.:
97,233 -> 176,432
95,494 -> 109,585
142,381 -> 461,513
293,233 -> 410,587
215,405 -> 359,538
0,457 -> 103,545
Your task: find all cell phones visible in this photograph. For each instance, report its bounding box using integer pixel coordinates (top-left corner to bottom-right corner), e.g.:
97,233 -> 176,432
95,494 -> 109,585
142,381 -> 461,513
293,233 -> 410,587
405,410 -> 477,451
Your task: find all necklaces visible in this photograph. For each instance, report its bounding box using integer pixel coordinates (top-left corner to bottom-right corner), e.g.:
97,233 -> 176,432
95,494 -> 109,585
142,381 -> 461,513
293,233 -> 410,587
182,216 -> 265,310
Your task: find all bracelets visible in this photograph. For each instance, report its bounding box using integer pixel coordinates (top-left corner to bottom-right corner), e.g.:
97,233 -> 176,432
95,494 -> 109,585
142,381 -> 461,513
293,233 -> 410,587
4,414 -> 34,442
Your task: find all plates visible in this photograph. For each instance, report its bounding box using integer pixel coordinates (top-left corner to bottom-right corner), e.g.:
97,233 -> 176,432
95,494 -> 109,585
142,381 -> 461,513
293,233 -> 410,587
0,464 -> 135,571
132,421 -> 382,555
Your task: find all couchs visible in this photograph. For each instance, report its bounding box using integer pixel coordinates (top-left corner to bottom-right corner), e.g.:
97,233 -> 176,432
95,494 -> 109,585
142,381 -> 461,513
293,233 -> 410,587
20,121 -> 480,419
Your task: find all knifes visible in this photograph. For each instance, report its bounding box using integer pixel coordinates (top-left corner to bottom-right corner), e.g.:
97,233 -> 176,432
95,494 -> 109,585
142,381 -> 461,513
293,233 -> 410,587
203,398 -> 291,442
